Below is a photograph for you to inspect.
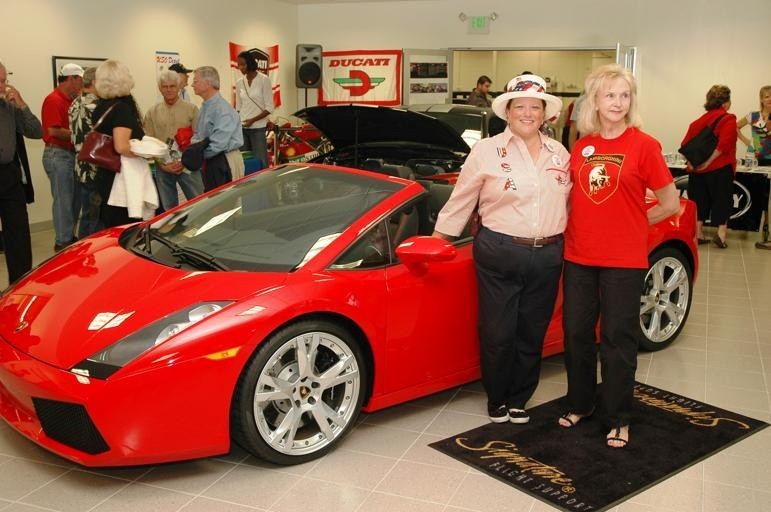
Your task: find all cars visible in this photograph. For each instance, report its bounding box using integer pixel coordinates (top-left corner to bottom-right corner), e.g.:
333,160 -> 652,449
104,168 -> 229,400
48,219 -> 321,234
393,104 -> 494,155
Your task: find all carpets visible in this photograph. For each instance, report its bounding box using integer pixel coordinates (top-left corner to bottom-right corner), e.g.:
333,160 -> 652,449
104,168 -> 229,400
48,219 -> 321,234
426,374 -> 771,512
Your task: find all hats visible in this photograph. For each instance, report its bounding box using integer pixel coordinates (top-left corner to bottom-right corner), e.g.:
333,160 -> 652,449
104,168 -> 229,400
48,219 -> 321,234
169,63 -> 194,75
490,73 -> 565,124
60,63 -> 86,78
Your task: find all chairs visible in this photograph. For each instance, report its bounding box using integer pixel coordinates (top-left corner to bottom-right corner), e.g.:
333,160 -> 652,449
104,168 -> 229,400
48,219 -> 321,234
423,182 -> 475,241
353,162 -> 422,262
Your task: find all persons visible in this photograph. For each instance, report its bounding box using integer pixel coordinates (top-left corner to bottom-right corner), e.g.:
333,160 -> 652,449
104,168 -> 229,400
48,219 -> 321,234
468,76 -> 492,108
432,74 -> 572,424
142,63 -> 245,213
1,64 -> 42,282
559,65 -> 680,449
41,58 -> 159,253
235,52 -> 273,169
735,85 -> 770,236
681,85 -> 737,248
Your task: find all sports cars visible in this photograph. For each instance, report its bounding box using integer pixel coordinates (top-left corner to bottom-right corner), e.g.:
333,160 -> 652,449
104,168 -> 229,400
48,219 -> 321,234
2,105 -> 702,469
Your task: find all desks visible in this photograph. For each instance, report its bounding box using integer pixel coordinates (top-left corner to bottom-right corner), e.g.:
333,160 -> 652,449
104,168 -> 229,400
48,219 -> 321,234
662,158 -> 771,243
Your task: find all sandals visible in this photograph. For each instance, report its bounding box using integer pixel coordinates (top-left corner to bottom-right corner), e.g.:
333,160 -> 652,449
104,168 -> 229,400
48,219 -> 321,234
605,421 -> 631,450
557,402 -> 598,428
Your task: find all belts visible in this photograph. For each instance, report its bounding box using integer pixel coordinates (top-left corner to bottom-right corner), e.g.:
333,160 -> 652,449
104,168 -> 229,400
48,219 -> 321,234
510,235 -> 560,248
44,142 -> 71,152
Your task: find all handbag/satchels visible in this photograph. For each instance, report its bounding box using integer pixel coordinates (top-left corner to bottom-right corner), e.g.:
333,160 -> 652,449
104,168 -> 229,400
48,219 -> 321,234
76,130 -> 129,173
21,182 -> 36,205
678,125 -> 718,170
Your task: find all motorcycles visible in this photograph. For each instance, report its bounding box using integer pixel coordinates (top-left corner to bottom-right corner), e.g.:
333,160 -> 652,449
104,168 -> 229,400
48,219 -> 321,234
266,117 -> 335,169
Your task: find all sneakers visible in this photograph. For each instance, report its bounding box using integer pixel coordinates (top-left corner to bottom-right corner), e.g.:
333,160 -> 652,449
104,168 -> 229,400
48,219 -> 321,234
755,241 -> 771,250
489,402 -> 510,423
509,407 -> 531,424
54,237 -> 81,253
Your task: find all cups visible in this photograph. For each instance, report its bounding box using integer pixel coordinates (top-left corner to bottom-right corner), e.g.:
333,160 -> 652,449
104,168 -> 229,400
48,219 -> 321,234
745,152 -> 759,171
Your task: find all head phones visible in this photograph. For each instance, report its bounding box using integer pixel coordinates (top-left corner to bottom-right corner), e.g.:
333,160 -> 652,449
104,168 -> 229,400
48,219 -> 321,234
244,53 -> 258,71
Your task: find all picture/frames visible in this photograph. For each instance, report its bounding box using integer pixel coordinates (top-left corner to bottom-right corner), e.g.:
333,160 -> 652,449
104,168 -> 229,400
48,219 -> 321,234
51,55 -> 108,90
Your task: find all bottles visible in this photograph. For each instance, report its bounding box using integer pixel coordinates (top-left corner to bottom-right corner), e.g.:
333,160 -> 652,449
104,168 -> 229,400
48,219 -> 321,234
664,153 -> 682,165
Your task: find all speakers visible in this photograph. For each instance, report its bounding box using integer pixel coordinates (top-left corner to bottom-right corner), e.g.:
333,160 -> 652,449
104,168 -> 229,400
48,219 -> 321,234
296,43 -> 323,88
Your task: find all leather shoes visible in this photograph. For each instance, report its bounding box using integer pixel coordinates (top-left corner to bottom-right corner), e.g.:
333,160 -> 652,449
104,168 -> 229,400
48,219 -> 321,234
713,233 -> 729,249
698,237 -> 712,245
